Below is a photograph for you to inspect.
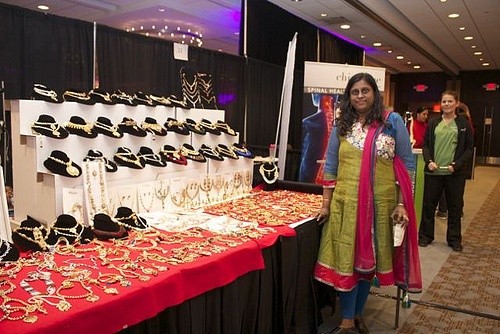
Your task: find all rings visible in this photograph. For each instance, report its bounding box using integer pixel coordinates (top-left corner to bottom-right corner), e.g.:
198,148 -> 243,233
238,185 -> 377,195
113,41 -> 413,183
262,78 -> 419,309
406,218 -> 409,221
403,215 -> 406,217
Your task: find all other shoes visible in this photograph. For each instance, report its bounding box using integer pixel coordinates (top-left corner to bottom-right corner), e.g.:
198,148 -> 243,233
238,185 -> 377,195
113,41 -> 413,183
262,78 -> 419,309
354,317 -> 369,334
448,243 -> 463,252
419,236 -> 432,247
437,211 -> 447,219
328,326 -> 359,334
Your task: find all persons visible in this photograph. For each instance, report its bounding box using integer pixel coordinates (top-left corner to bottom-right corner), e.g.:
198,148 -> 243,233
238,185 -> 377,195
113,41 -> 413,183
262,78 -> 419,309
406,90 -> 475,252
298,93 -> 327,182
315,74 -> 422,334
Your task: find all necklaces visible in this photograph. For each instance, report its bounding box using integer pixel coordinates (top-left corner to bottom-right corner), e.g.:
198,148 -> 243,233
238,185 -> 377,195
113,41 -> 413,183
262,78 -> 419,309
0,73 -> 323,326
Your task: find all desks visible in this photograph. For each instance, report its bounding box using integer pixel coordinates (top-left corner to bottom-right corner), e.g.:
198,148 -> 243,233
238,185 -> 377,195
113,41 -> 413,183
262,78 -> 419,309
0,188 -> 330,334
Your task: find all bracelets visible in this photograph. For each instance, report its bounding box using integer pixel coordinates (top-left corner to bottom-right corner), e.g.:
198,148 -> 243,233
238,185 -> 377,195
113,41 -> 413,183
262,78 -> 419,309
323,199 -> 331,202
397,203 -> 404,206
